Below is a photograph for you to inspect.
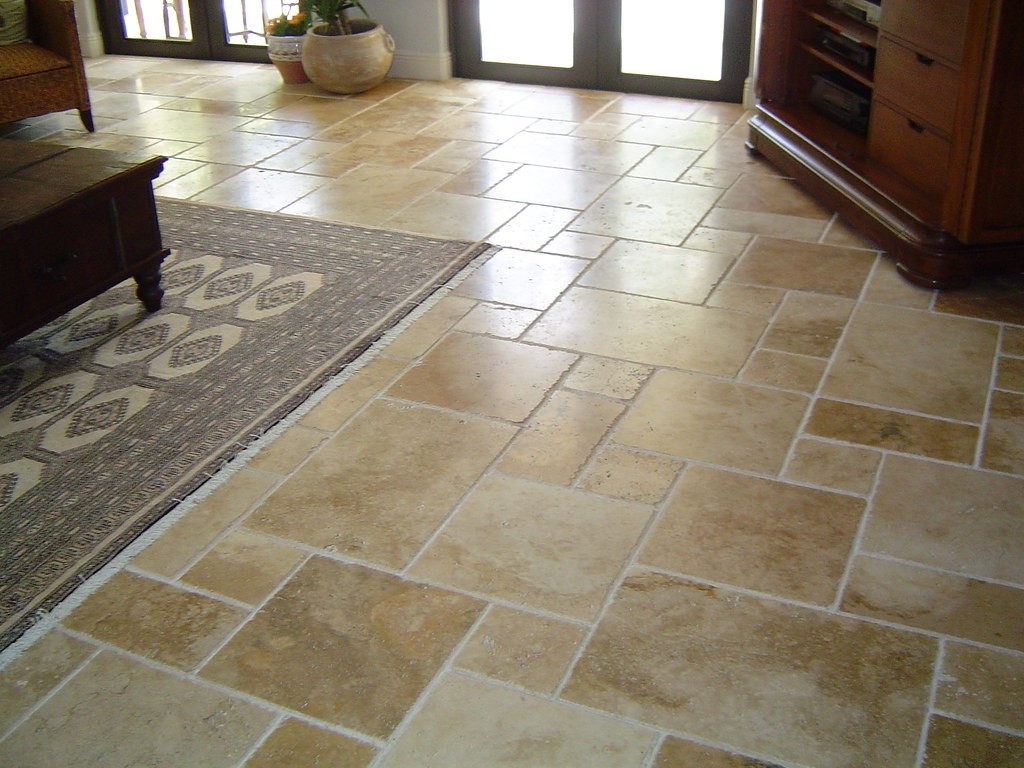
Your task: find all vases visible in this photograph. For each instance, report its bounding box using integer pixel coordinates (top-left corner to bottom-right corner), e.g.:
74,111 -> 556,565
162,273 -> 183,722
267,33 -> 310,84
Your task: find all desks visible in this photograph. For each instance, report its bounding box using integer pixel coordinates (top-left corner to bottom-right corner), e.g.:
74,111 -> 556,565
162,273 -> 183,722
0,134 -> 172,350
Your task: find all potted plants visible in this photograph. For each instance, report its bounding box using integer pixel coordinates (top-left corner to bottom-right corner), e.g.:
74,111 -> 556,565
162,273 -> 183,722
297,0 -> 395,94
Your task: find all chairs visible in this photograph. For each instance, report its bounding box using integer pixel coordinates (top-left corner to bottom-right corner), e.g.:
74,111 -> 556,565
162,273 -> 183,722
0,0 -> 96,133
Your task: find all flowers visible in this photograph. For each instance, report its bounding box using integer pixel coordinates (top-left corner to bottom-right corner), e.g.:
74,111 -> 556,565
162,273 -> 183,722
262,12 -> 311,35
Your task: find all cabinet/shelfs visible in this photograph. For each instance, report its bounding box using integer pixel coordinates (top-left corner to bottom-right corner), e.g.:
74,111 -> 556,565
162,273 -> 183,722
742,0 -> 1023,288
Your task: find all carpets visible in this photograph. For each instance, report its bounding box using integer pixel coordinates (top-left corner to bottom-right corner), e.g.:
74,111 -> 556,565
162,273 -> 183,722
0,189 -> 504,669
